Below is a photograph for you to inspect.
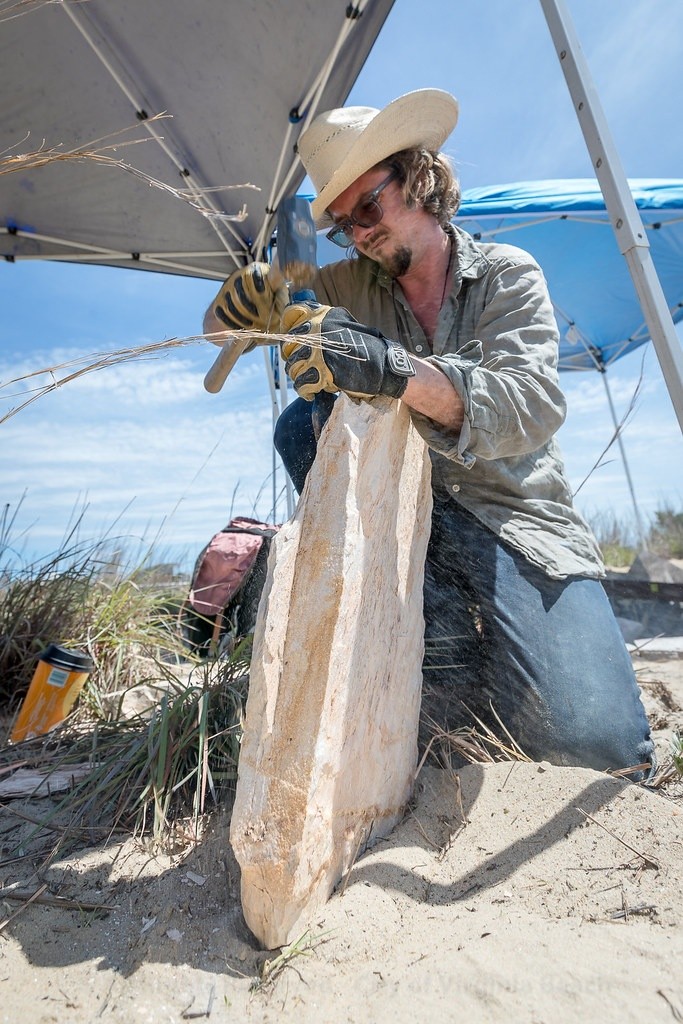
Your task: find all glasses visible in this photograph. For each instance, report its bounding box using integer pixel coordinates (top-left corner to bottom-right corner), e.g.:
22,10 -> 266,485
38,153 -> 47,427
325,169 -> 403,249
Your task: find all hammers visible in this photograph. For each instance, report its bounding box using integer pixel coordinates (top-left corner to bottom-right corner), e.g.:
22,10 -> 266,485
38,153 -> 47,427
203,197 -> 320,394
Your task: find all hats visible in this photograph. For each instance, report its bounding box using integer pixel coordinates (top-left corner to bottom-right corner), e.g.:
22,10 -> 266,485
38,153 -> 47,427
297,87 -> 459,231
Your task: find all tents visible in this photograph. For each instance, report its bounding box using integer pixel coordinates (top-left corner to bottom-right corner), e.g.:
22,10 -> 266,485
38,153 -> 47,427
0,0 -> 683,554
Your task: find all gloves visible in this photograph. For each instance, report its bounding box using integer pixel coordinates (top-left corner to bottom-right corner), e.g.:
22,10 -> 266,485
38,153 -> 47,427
214,262 -> 290,344
281,303 -> 415,405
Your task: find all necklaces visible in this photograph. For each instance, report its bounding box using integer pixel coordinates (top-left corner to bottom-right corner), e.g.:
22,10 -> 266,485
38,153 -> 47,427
392,233 -> 452,339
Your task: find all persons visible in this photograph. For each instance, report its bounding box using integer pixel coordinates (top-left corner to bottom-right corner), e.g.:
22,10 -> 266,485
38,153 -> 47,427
203,87 -> 656,781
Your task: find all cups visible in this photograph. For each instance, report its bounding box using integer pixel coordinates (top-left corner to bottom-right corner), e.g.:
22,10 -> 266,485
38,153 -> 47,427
12,644 -> 95,742
